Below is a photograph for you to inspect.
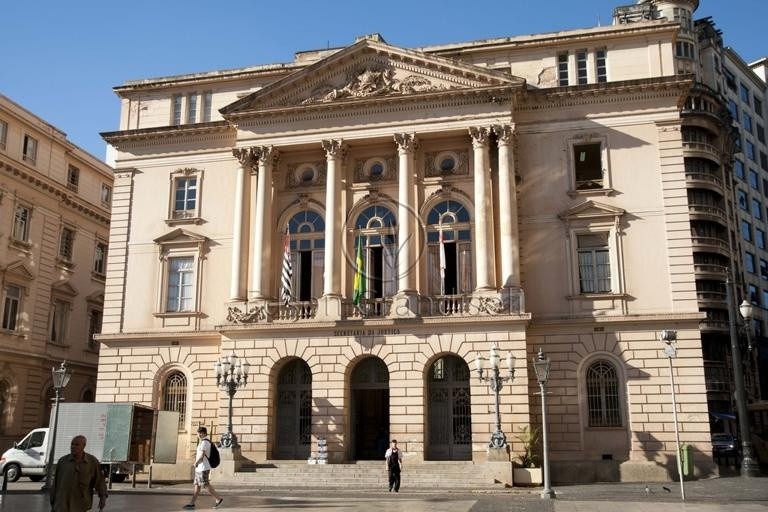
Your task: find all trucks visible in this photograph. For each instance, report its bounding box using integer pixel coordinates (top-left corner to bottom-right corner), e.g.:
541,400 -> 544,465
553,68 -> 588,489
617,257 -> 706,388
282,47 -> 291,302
0,400 -> 180,482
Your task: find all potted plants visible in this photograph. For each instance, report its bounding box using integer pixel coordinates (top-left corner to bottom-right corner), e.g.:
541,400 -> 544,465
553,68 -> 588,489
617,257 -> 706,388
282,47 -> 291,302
512,425 -> 543,486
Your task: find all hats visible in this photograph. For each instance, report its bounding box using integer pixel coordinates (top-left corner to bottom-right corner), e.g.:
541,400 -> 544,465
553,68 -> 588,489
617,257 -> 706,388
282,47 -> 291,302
197,427 -> 206,432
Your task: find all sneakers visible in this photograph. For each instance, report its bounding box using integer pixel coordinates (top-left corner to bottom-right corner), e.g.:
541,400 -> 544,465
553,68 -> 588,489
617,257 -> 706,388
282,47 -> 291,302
212,498 -> 223,509
183,504 -> 195,509
389,488 -> 398,492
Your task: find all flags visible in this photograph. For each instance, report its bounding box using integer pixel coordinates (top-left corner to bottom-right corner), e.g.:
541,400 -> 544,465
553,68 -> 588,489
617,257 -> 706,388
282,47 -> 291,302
279,233 -> 293,309
351,233 -> 366,306
436,212 -> 448,281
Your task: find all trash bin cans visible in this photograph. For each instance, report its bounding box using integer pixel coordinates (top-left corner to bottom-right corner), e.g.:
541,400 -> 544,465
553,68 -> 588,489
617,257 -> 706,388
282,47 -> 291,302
680,444 -> 693,479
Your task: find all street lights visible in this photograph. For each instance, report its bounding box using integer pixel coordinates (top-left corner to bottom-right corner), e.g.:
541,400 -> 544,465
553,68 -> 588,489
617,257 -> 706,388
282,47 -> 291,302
217,350 -> 250,448
723,265 -> 758,476
532,347 -> 551,492
475,339 -> 515,448
660,329 -> 686,501
42,360 -> 73,488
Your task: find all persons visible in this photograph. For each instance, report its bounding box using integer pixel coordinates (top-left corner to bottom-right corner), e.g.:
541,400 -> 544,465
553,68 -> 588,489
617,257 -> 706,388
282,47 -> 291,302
181,426 -> 225,510
47,434 -> 108,512
383,439 -> 404,493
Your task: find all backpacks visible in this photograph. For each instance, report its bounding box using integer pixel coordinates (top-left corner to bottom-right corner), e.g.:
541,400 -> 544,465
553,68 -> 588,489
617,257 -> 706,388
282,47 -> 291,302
203,439 -> 220,468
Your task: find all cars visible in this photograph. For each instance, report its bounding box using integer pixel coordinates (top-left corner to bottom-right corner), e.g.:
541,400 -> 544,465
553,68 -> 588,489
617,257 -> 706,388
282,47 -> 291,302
711,432 -> 737,453
